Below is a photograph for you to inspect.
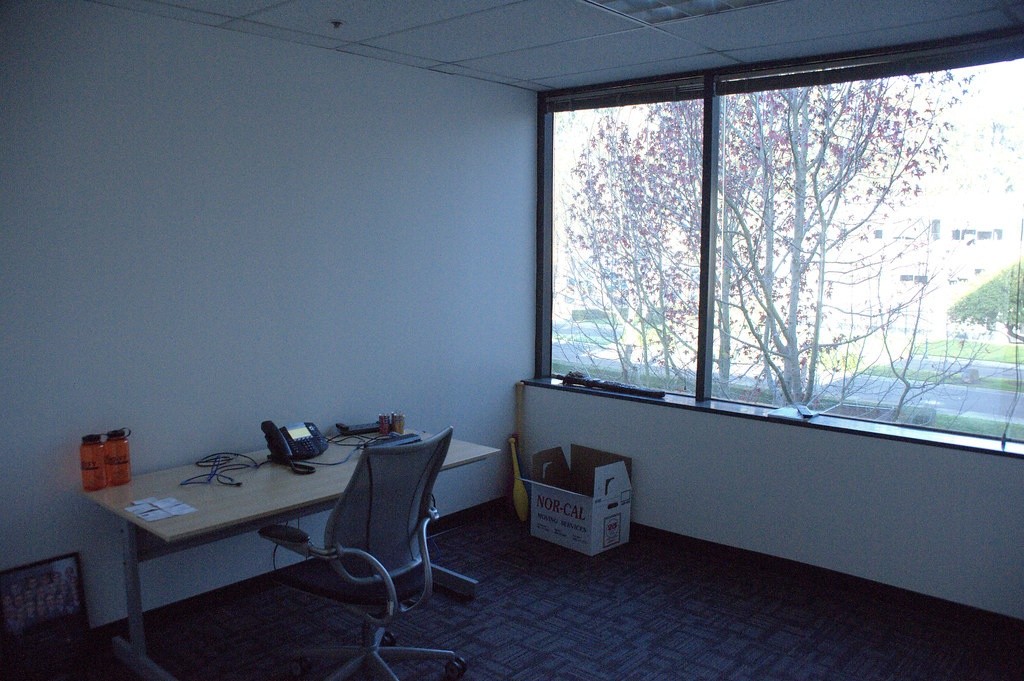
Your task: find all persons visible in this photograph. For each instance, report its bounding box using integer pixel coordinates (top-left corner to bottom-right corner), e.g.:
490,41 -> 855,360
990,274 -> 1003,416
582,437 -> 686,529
2,567 -> 80,631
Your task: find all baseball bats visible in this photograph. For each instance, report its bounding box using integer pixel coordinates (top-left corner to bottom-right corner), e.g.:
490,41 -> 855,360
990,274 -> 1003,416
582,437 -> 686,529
517,382 -> 525,455
508,437 -> 529,522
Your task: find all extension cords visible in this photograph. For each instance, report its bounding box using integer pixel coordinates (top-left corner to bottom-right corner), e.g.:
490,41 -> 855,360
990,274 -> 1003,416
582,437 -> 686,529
337,422 -> 380,435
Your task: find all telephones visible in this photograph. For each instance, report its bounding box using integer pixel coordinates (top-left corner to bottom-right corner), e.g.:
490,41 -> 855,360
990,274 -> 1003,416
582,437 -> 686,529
261,420 -> 329,461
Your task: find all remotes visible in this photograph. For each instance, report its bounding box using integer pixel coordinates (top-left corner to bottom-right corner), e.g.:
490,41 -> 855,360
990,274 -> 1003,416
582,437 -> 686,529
797,405 -> 813,418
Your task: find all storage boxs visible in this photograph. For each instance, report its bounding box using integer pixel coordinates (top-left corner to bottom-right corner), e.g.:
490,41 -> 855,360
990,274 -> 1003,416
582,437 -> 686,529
514,442 -> 633,556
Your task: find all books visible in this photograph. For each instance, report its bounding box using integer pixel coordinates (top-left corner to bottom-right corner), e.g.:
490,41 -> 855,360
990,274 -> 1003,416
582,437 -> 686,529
768,408 -> 819,422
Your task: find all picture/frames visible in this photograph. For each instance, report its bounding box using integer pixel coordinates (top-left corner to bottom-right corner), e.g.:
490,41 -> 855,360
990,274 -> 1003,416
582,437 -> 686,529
0,551 -> 90,640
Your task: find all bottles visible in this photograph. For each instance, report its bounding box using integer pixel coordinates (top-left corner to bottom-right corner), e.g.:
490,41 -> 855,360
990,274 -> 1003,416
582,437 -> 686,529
80,433 -> 109,490
104,427 -> 132,485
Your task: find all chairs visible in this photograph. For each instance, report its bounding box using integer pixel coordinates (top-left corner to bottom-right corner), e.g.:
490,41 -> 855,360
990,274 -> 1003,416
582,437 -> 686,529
258,425 -> 467,681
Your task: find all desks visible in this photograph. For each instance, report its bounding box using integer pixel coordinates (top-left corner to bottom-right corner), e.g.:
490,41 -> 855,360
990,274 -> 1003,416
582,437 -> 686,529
82,429 -> 502,681
